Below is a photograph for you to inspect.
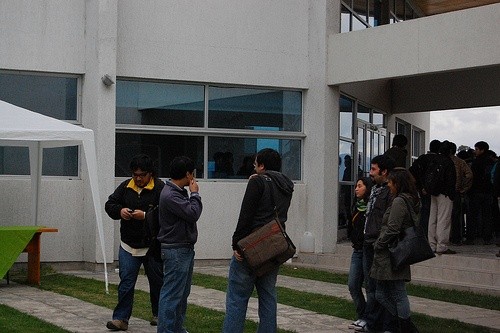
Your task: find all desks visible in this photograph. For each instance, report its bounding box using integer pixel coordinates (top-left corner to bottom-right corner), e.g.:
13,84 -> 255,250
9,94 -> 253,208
0,225 -> 58,286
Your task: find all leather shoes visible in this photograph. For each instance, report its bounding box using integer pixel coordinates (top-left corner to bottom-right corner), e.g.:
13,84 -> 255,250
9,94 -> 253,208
434,250 -> 437,253
438,248 -> 457,254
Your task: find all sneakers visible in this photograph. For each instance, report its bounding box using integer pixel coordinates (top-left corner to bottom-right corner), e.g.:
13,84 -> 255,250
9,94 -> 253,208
354,321 -> 368,330
106,319 -> 128,331
151,317 -> 158,326
348,318 -> 362,329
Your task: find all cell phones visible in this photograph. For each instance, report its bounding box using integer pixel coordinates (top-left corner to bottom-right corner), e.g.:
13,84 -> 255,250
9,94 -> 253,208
128,209 -> 132,213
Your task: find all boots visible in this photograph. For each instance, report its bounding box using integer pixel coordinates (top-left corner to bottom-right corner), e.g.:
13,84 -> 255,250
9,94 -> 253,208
392,314 -> 419,333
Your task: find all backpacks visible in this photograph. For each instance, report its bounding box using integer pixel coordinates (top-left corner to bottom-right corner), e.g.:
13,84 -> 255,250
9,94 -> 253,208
426,154 -> 457,197
409,153 -> 430,187
147,201 -> 160,248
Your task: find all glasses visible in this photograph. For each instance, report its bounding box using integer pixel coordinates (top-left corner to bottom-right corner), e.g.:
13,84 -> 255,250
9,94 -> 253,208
253,163 -> 256,166
131,170 -> 150,178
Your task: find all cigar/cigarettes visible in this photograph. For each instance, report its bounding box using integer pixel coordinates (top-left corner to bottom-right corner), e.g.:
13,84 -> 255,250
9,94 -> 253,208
195,180 -> 199,183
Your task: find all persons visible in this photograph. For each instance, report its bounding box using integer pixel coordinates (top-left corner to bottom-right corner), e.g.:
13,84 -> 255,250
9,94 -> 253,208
346,153 -> 424,333
224,148 -> 294,333
406,140 -> 500,255
157,156 -> 202,333
105,154 -> 165,330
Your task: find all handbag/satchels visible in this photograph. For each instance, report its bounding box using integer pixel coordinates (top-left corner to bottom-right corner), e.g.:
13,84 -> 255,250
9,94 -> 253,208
236,218 -> 296,280
386,227 -> 437,271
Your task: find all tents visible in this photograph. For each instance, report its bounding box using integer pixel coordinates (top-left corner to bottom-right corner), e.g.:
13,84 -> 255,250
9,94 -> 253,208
0,99 -> 110,296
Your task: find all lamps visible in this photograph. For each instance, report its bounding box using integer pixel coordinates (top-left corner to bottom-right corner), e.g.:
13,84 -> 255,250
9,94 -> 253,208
101,73 -> 114,87
